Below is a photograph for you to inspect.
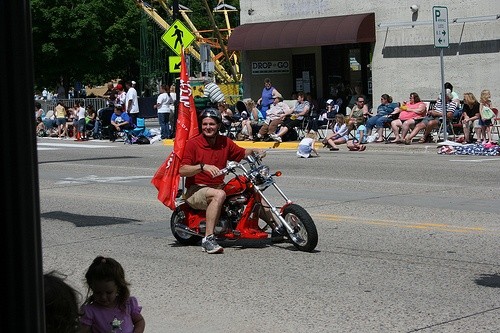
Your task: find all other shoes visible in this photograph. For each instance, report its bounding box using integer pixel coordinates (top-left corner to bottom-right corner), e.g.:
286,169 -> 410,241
270,134 -> 282,142
252,136 -> 264,140
462,141 -> 470,144
377,137 -> 383,142
330,148 -> 339,151
418,137 -> 426,143
391,139 -> 400,142
397,139 -> 411,145
475,140 -> 482,144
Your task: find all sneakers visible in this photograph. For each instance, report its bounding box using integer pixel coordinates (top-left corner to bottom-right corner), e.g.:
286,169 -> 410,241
202,233 -> 223,254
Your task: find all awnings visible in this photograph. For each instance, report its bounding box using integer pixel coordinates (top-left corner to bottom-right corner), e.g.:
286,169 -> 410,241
228,12 -> 376,50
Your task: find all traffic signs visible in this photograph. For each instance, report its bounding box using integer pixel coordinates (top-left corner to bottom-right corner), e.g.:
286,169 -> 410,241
432,6 -> 451,49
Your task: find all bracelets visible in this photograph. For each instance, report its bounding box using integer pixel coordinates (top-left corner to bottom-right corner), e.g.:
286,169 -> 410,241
201,164 -> 204,171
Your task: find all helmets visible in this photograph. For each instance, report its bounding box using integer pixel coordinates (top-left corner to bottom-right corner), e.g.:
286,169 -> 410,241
199,109 -> 222,119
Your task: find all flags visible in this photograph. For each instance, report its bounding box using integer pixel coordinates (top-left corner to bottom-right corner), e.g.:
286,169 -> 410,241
150,50 -> 199,210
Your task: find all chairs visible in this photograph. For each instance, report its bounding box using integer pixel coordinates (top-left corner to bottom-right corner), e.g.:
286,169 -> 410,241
296,104 -> 500,143
75,109 -> 146,143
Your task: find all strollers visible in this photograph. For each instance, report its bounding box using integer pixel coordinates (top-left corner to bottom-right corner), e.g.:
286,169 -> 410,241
88,106 -> 118,141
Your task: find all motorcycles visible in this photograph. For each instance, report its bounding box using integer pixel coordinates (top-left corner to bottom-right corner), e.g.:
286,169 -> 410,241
170,146 -> 320,251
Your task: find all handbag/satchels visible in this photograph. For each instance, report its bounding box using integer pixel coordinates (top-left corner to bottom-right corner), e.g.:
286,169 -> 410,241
481,105 -> 495,121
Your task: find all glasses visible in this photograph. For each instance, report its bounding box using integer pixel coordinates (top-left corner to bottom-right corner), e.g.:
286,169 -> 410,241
358,101 -> 365,103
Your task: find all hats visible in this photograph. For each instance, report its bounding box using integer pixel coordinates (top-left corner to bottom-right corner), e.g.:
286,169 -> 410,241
325,99 -> 334,105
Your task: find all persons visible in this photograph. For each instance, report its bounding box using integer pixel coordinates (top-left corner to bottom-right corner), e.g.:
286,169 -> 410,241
178,108 -> 266,253
42,256 -> 145,333
366,94 -> 400,142
199,77 -> 224,106
218,91 -> 319,143
390,92 -> 426,142
439,82 -> 459,103
153,83 -> 177,139
458,92 -> 483,143
407,89 -> 457,143
297,85 -> 369,158
257,78 -> 283,119
34,80 -> 139,142
479,90 -> 494,146
